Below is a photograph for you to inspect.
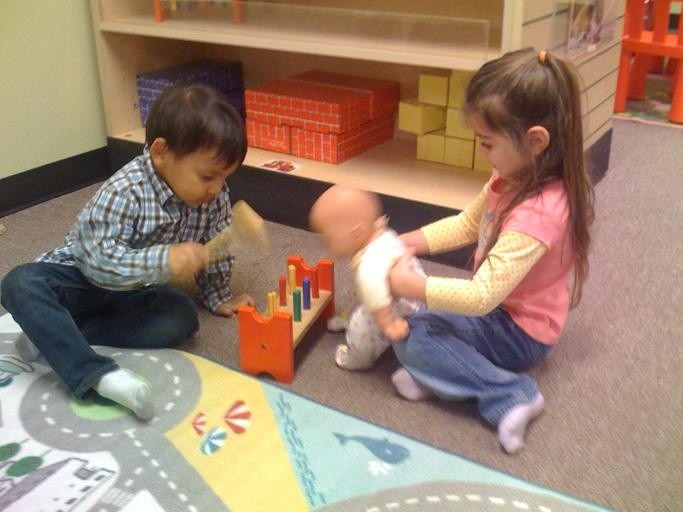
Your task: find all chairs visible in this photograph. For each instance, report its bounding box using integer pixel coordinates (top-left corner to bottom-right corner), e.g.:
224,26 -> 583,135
614,0 -> 683,123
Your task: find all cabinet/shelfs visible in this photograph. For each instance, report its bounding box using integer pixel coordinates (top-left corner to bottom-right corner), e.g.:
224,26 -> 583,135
87,0 -> 625,268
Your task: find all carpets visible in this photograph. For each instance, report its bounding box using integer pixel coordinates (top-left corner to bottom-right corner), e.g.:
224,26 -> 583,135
0,311 -> 621,512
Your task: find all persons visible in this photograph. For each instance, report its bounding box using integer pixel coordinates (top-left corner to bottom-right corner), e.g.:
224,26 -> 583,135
385,46 -> 596,456
308,182 -> 427,372
0,83 -> 258,421
